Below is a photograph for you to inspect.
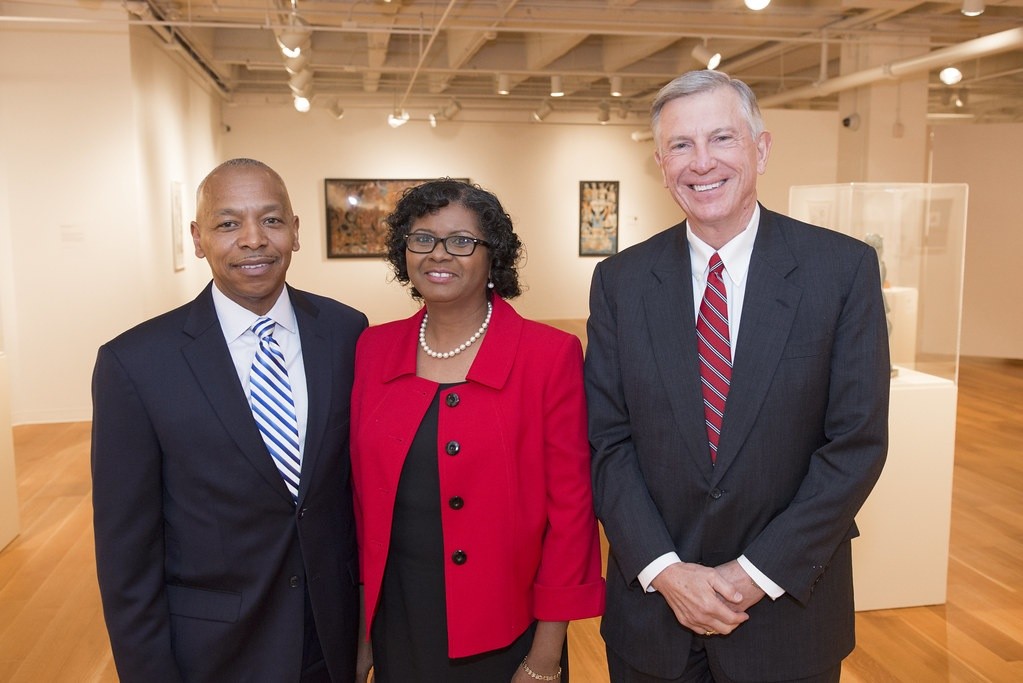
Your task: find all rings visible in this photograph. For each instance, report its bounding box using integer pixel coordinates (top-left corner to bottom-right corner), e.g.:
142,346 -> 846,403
705,631 -> 715,636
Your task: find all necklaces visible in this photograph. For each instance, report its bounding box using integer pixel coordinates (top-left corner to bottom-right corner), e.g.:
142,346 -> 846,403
419,300 -> 492,359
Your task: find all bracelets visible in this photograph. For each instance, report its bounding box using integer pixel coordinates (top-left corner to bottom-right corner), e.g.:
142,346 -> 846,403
522,656 -> 561,681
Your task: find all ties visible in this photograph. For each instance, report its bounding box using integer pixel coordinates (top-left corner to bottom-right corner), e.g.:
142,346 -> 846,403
247,319 -> 302,507
695,253 -> 734,470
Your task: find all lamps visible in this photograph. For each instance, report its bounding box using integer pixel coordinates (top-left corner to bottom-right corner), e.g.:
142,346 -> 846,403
270,0 -> 984,143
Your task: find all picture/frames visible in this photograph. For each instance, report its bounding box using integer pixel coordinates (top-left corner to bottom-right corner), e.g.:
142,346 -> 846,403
169,178 -> 190,271
578,181 -> 620,258
320,176 -> 471,264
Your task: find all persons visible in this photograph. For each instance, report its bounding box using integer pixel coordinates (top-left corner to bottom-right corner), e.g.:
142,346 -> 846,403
348,178 -> 607,683
90,158 -> 368,683
582,69 -> 891,683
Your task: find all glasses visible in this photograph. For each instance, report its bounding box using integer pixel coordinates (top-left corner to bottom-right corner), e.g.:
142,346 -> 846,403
401,232 -> 492,257
